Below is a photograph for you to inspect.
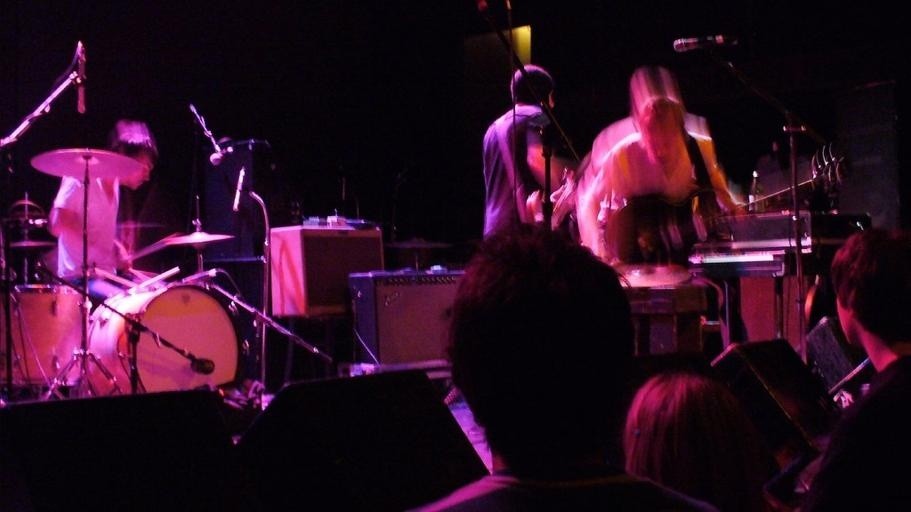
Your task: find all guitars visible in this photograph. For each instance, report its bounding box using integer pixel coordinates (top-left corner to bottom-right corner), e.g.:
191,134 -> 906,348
527,187 -> 579,229
621,144 -> 862,266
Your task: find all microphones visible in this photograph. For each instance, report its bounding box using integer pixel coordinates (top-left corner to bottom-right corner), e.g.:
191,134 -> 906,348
670,32 -> 724,55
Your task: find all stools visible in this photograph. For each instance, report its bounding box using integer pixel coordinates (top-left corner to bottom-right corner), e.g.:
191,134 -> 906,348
625,286 -> 707,358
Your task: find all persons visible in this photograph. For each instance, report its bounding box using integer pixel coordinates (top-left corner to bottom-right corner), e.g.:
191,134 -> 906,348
626,361 -> 770,512
415,222 -> 721,512
47,119 -> 167,316
793,223 -> 911,511
480,65 -> 578,244
573,66 -> 752,348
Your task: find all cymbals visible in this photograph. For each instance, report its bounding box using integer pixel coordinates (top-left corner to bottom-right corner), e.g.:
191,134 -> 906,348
165,233 -> 234,245
30,148 -> 146,182
116,221 -> 163,229
383,236 -> 452,250
9,240 -> 54,248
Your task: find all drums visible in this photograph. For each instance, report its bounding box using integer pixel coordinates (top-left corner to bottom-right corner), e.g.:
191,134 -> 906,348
81,282 -> 240,398
0,283 -> 90,387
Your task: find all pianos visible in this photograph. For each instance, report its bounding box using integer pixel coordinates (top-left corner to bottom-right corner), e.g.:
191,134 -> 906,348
688,211 -> 870,277
